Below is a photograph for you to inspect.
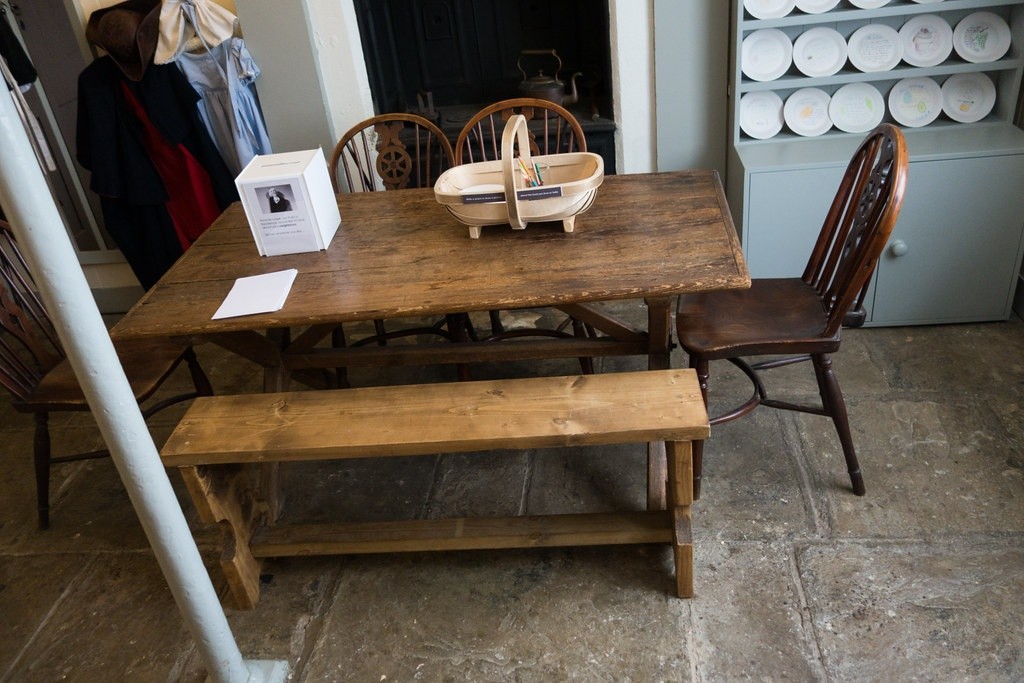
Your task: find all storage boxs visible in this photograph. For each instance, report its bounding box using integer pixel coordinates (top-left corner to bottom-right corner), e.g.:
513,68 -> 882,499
234,146 -> 340,258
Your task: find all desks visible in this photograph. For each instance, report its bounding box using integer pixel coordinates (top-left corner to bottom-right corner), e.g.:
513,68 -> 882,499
111,169 -> 751,510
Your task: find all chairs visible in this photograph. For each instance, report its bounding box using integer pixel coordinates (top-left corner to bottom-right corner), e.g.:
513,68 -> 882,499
674,124 -> 907,501
328,113 -> 477,392
1,218 -> 216,530
456,98 -> 599,377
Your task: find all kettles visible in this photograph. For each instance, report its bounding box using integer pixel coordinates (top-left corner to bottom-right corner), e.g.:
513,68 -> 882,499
518,48 -> 582,120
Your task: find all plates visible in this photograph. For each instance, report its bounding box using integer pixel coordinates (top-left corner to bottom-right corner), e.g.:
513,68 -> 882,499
741,28 -> 793,82
793,26 -> 848,78
744,0 -> 945,19
739,90 -> 785,140
953,12 -> 1012,63
848,24 -> 903,74
899,14 -> 953,68
888,77 -> 944,128
828,82 -> 885,134
784,87 -> 834,136
942,70 -> 997,124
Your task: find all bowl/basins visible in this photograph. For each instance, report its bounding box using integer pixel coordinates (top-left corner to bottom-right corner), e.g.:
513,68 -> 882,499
459,184 -> 504,194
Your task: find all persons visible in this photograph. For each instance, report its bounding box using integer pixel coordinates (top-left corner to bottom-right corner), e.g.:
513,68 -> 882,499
267,187 -> 291,213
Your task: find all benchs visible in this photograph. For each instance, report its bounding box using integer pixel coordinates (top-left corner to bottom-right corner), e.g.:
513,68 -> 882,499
159,366 -> 712,607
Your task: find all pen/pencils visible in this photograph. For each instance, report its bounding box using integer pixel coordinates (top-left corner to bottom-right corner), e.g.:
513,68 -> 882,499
535,163 -> 544,186
516,159 -> 533,187
530,159 -> 540,185
518,156 -> 537,187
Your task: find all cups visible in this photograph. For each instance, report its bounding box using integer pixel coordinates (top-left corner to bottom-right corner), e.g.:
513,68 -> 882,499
521,162 -> 551,188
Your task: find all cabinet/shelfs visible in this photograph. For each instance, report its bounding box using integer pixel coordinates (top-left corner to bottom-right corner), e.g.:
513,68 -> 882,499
722,0 -> 1024,327
353,0 -> 615,191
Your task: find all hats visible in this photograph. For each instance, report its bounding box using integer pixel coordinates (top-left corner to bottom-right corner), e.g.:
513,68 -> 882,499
85,0 -> 162,81
